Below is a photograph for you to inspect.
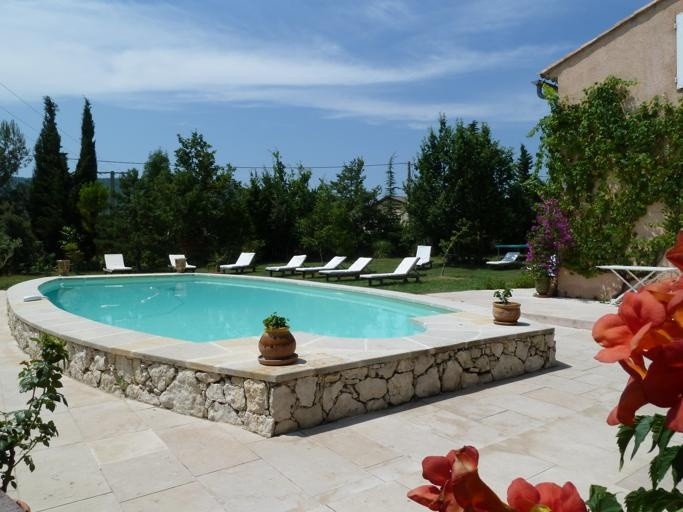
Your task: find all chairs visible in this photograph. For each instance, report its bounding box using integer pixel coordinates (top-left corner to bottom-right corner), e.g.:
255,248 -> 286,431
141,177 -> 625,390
217,252 -> 258,273
358,256 -> 420,286
318,257 -> 373,281
103,253 -> 133,274
411,246 -> 433,284
485,252 -> 520,270
295,256 -> 348,278
264,253 -> 308,277
167,253 -> 198,272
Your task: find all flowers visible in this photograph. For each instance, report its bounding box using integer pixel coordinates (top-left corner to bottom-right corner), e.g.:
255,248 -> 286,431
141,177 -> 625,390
405,229 -> 683,512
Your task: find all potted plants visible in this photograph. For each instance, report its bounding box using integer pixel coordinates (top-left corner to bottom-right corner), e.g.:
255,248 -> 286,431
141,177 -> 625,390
257,312 -> 298,366
527,261 -> 554,294
493,286 -> 521,325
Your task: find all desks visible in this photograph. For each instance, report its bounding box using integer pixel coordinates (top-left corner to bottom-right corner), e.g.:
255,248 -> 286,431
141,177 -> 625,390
596,265 -> 679,305
